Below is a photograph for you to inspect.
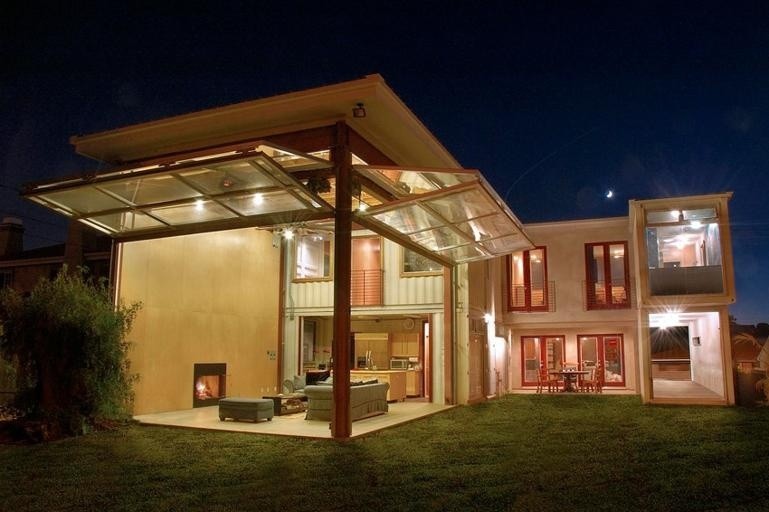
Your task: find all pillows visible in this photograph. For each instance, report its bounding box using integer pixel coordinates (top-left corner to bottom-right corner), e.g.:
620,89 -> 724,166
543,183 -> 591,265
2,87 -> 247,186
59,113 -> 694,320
293,375 -> 304,389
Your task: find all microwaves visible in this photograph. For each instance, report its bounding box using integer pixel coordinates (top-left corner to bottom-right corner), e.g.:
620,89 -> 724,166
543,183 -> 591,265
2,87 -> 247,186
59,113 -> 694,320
389,360 -> 409,370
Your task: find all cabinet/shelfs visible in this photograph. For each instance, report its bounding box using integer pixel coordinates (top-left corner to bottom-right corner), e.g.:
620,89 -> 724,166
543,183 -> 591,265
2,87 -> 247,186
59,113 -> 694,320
405,369 -> 423,399
391,332 -> 422,359
547,342 -> 555,369
335,371 -> 406,402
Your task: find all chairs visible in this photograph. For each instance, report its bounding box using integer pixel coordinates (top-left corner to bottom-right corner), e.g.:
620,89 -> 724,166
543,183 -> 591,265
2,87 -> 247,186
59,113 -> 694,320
578,363 -> 604,395
563,361 -> 583,393
543,368 -> 561,393
535,365 -> 559,393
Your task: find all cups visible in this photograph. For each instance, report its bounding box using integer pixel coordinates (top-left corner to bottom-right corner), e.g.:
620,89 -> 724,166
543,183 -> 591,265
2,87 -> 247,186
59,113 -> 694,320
562,368 -> 574,373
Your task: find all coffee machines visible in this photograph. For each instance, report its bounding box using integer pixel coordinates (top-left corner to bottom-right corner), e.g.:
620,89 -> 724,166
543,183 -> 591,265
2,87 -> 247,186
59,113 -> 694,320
408,357 -> 419,370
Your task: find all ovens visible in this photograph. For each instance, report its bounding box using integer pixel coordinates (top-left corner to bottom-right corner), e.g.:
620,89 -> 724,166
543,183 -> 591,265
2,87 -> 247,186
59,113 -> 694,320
357,356 -> 370,369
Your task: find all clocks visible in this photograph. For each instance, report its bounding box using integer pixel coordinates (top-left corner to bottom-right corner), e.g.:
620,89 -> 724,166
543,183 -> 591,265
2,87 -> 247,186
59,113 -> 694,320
404,317 -> 415,331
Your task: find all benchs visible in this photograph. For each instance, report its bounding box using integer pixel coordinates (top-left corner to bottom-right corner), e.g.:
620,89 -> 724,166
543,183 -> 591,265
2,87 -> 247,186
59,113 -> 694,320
217,399 -> 276,424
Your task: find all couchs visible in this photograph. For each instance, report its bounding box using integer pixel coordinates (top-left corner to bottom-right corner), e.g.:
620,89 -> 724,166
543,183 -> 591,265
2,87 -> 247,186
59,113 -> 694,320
283,373 -> 305,391
301,379 -> 392,423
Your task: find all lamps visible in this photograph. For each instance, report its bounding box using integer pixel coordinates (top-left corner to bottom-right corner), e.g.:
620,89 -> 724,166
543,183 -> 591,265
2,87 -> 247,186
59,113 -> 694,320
352,102 -> 366,119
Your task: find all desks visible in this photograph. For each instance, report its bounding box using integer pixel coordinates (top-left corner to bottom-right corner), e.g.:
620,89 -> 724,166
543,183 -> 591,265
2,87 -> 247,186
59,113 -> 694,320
548,370 -> 587,393
